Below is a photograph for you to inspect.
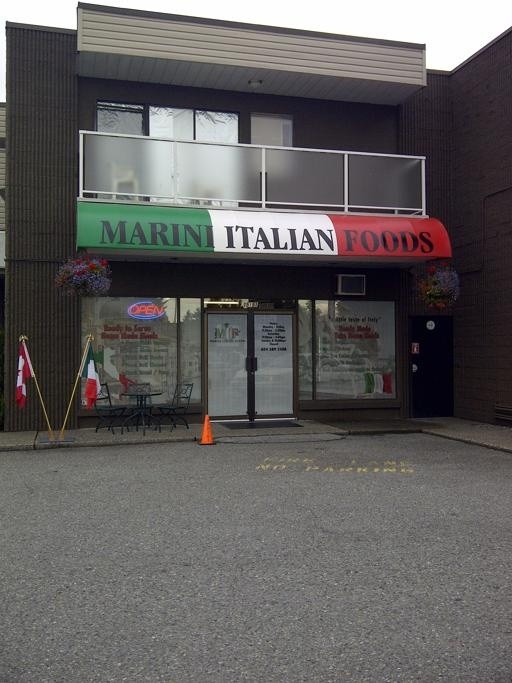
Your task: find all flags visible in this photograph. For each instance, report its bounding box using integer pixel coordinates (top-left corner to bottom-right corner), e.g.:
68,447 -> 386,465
15,333 -> 36,413
77,332 -> 103,415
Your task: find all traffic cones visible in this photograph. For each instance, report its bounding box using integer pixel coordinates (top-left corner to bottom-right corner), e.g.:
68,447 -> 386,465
201,414 -> 213,444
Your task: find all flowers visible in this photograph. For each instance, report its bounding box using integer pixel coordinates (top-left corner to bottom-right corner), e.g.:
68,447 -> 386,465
54,250 -> 113,298
416,260 -> 460,313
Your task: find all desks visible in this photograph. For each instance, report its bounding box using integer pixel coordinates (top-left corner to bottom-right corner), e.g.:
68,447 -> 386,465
119,392 -> 163,436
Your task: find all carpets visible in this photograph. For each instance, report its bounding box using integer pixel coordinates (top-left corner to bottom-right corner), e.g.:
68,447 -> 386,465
219,420 -> 304,430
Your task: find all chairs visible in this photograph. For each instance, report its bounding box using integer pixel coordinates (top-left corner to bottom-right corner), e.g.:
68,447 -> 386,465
155,383 -> 194,432
127,382 -> 156,431
93,383 -> 130,434
